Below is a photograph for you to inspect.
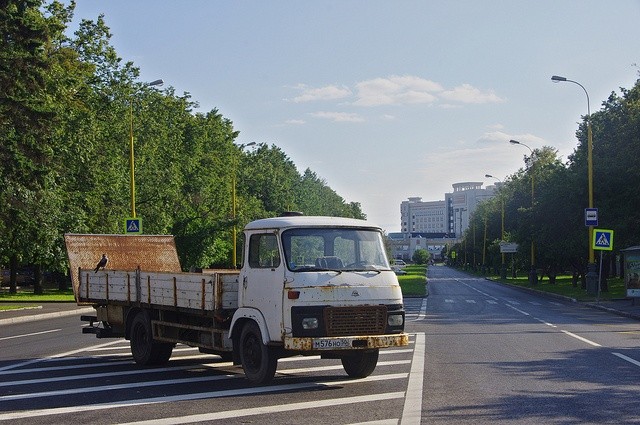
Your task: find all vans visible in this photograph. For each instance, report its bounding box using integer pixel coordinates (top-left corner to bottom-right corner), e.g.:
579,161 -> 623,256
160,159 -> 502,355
395,260 -> 407,266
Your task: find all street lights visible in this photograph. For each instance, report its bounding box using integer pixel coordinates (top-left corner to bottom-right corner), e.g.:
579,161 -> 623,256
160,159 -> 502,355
510,139 -> 538,283
485,174 -> 507,280
551,75 -> 599,294
463,208 -> 477,272
232,141 -> 257,266
129,78 -> 165,217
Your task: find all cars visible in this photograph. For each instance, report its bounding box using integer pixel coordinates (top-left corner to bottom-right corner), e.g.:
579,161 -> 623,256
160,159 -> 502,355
390,265 -> 407,276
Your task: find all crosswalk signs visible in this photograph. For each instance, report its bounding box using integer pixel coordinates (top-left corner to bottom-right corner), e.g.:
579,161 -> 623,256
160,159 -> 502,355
123,217 -> 144,235
592,229 -> 615,250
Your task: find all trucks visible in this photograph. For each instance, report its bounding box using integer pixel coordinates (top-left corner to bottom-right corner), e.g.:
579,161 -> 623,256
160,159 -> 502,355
63,211 -> 409,386
